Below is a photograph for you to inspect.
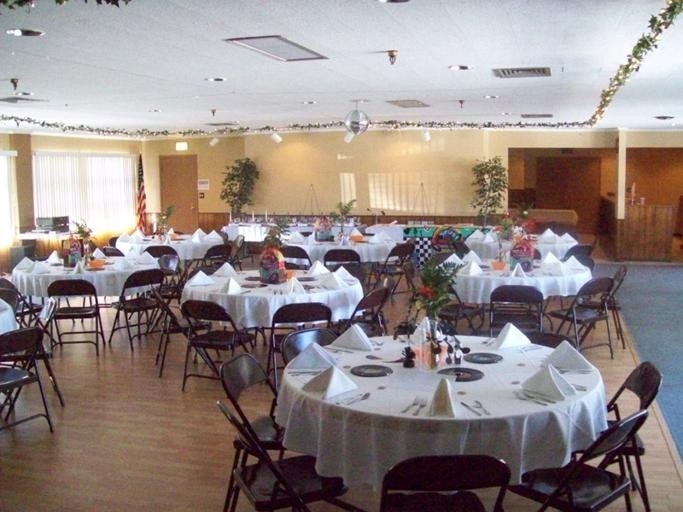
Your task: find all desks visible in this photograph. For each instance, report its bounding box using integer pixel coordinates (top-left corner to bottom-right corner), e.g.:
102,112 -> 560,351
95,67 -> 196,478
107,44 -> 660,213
181,266 -> 360,327
444,258 -> 593,300
222,220 -> 361,257
1,299 -> 13,332
13,254 -> 157,297
365,223 -> 405,234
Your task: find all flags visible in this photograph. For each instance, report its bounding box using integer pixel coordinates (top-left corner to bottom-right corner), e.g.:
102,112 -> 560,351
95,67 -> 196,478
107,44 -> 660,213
135,156 -> 146,234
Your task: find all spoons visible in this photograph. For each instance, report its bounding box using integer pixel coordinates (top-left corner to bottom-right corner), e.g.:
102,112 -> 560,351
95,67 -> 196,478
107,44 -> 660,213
346,392 -> 369,406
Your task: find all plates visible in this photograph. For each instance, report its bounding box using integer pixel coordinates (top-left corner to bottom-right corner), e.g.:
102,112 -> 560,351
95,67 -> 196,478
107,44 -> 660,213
298,277 -> 318,281
351,365 -> 394,377
240,284 -> 268,289
438,368 -> 484,382
464,352 -> 503,364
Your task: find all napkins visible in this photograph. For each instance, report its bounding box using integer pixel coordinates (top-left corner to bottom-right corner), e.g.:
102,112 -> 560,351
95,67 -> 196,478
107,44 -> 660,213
33,262 -> 48,275
219,277 -> 240,295
314,274 -> 347,291
542,340 -> 592,371
491,324 -> 522,349
46,251 -> 62,264
323,368 -> 359,401
15,255 -> 33,272
186,270 -> 214,288
73,260 -> 85,275
279,277 -> 304,295
331,324 -> 375,352
428,377 -> 454,418
524,366 -> 576,401
288,344 -> 337,369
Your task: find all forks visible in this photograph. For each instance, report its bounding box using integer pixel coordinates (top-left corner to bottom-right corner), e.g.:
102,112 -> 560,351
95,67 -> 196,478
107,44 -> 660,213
512,391 -> 548,407
400,397 -> 428,416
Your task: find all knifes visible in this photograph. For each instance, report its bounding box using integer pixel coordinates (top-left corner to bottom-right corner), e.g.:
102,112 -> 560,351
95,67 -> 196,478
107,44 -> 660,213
460,402 -> 481,416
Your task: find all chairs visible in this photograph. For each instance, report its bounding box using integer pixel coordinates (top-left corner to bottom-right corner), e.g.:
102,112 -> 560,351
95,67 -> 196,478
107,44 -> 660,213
379,451 -> 510,510
4,326 -> 56,434
440,281 -> 481,336
108,270 -> 165,351
147,295 -> 209,374
572,360 -> 662,505
266,303 -> 334,389
282,327 -> 337,364
584,266 -> 627,349
219,353 -> 298,502
341,287 -> 392,339
181,297 -> 256,398
2,277 -> 44,326
8,299 -> 67,405
552,277 -> 614,360
524,330 -> 577,349
501,408 -> 647,511
489,282 -> 543,335
49,279 -> 105,356
212,400 -> 352,512
88,231 -> 602,282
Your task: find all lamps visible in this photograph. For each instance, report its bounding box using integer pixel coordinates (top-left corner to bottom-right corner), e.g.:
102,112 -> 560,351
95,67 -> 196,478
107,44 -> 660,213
423,132 -> 431,142
210,138 -> 219,146
271,134 -> 282,143
345,132 -> 355,143
